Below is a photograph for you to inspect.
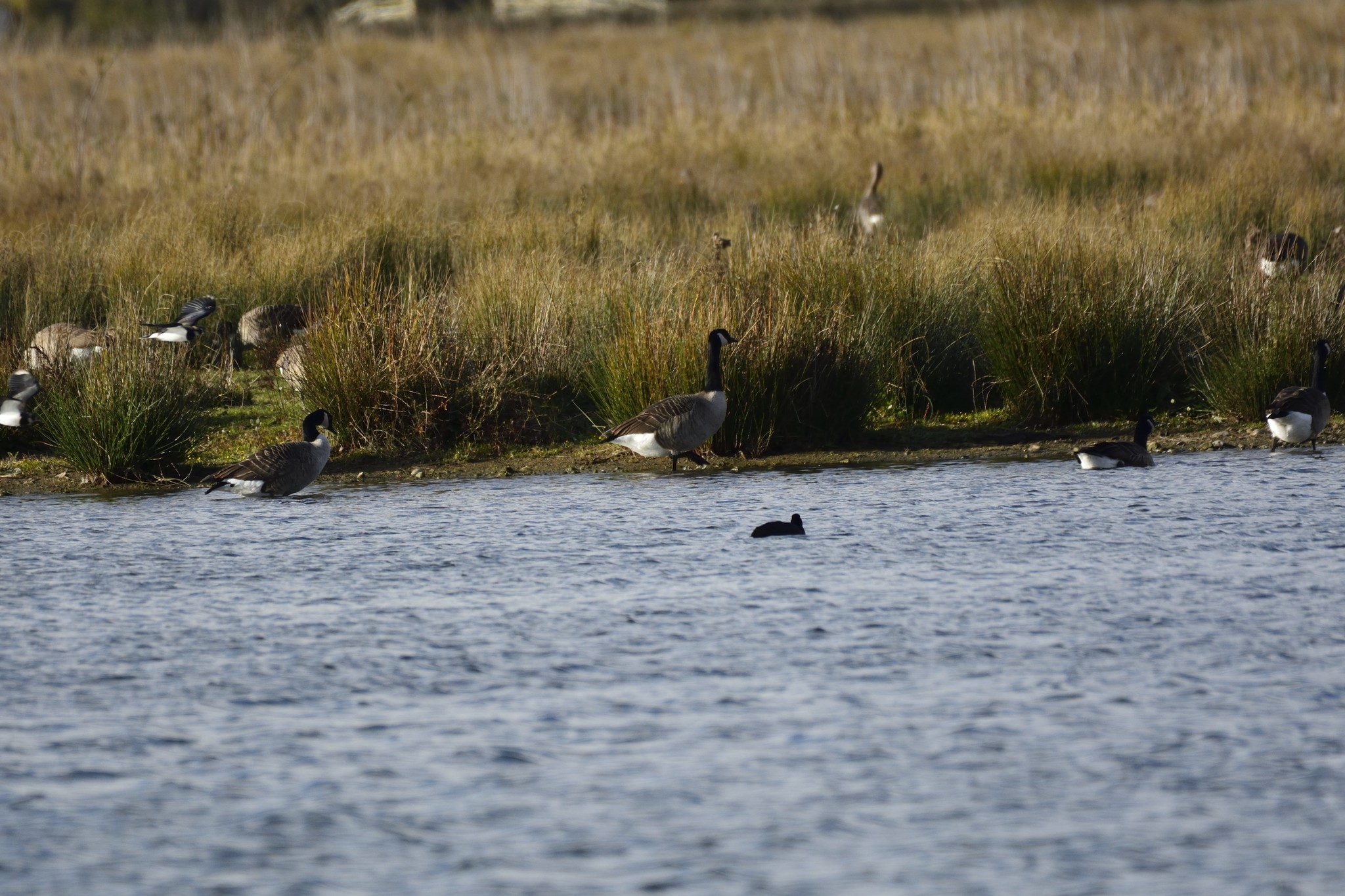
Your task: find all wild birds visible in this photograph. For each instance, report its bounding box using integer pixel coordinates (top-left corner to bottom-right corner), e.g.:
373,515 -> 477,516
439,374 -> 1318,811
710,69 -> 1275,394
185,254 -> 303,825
749,514 -> 806,539
199,409 -> 337,499
1260,232 -> 1309,285
134,294 -> 216,342
690,233 -> 725,288
859,161 -> 886,235
1264,339 -> 1331,453
237,304 -> 323,398
0,369 -> 43,427
1073,414 -> 1160,470
23,323 -> 116,370
598,329 -> 739,471
